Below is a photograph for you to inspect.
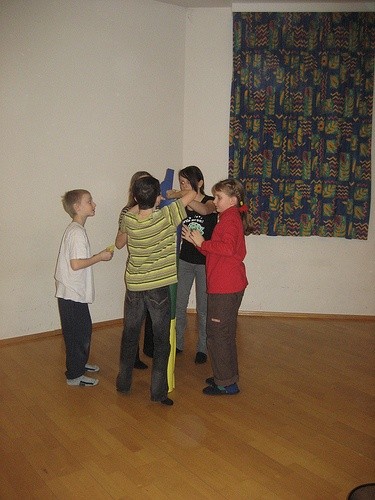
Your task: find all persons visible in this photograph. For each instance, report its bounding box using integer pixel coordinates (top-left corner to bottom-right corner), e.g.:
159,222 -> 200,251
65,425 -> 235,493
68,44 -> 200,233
55,189 -> 115,387
180,178 -> 251,396
118,170 -> 161,371
116,177 -> 198,407
174,166 -> 221,365
